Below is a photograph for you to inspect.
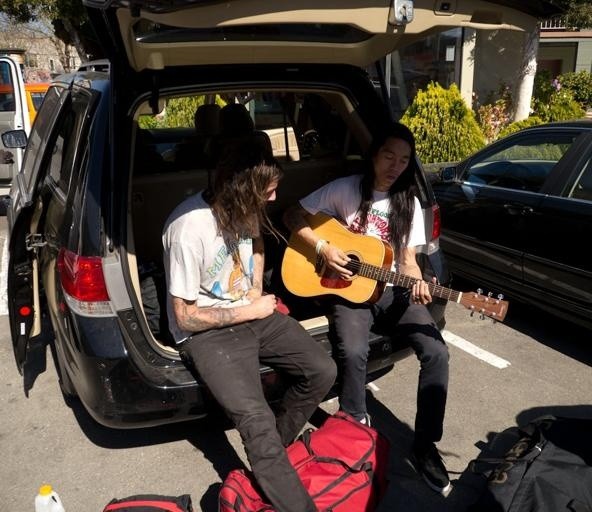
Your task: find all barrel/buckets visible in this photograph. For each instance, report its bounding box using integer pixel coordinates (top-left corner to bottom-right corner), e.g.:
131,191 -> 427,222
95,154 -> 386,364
34,485 -> 65,512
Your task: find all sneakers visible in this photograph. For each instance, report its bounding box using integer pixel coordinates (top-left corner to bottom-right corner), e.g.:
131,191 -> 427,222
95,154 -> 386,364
403,440 -> 451,492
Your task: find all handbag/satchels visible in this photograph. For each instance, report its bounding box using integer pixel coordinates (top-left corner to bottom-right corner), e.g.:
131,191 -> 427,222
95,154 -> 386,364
217,409 -> 391,512
441,417 -> 592,512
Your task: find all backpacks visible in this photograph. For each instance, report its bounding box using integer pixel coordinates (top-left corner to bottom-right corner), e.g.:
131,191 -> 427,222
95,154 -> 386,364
103,493 -> 192,512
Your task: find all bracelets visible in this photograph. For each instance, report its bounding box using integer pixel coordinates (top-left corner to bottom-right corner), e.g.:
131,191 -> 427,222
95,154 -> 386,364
316,240 -> 326,255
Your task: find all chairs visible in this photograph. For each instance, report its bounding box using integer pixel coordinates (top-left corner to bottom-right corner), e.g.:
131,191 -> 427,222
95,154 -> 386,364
204,102 -> 270,164
171,103 -> 221,169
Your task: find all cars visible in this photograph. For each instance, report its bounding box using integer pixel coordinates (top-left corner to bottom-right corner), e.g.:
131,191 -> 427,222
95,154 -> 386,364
420,118 -> 591,333
0,56 -> 31,217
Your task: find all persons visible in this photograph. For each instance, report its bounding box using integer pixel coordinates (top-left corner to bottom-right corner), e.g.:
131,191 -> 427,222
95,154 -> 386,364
158,146 -> 339,512
193,101 -> 222,132
551,71 -> 562,93
282,126 -> 450,492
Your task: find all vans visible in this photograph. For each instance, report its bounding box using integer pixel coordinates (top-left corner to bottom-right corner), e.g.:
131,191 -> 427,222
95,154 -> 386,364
2,1 -> 571,431
25,83 -> 51,132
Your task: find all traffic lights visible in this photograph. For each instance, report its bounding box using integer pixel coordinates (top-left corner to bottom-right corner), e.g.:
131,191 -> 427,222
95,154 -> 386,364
49,59 -> 55,72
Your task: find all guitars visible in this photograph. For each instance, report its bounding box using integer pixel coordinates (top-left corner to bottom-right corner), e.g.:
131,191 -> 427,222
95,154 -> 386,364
281,211 -> 508,324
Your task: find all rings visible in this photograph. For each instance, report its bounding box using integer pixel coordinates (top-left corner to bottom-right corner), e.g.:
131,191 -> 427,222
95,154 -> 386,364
416,297 -> 420,301
